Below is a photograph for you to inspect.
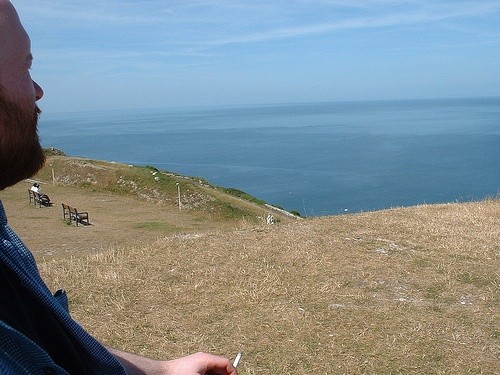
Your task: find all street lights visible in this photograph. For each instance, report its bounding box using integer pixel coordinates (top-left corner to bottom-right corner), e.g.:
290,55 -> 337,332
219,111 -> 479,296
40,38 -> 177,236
49,164 -> 55,186
175,182 -> 182,213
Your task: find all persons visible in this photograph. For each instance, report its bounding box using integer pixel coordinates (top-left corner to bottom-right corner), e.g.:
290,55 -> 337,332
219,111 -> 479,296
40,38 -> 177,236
31,182 -> 41,197
0,0 -> 238,375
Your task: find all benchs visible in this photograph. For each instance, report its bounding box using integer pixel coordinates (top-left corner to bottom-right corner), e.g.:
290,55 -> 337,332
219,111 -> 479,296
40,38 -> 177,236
28,188 -> 50,208
61,203 -> 88,227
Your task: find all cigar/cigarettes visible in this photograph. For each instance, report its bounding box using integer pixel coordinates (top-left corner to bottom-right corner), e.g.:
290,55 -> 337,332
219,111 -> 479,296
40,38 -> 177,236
233,351 -> 243,368
